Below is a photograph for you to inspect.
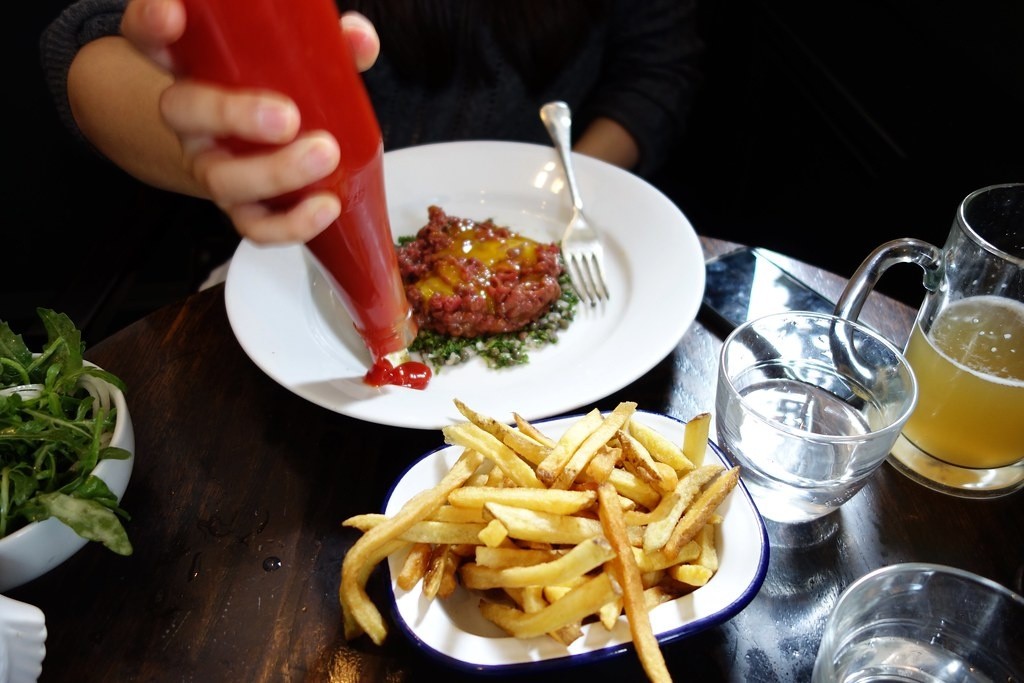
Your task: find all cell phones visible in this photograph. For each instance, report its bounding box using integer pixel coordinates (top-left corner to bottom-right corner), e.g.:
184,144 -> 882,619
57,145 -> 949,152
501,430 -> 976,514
701,246 -> 903,407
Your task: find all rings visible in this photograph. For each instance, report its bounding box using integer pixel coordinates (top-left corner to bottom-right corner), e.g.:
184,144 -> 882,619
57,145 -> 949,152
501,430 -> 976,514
159,84 -> 175,118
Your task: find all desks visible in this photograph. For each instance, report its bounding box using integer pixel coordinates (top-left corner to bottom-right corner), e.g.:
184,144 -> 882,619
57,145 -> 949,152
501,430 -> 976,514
0,233 -> 1023,683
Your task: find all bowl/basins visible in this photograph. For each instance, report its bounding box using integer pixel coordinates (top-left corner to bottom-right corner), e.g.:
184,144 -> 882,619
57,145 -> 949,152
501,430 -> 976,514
0,349 -> 133,591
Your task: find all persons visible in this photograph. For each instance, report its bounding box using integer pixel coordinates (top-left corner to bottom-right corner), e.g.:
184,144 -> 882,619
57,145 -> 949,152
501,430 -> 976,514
37,0 -> 704,249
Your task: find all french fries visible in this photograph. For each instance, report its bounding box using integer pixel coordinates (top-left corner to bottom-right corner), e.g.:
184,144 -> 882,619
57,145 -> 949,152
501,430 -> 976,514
339,398 -> 739,683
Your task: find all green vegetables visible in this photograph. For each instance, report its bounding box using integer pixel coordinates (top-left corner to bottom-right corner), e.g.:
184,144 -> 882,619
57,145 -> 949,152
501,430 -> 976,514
389,232 -> 577,366
0,308 -> 134,559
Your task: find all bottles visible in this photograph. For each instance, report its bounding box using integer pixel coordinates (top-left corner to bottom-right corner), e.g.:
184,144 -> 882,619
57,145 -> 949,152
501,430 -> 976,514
150,0 -> 420,357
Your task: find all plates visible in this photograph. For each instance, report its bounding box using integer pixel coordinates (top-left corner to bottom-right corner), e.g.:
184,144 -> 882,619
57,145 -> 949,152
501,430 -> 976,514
225,138 -> 707,427
385,407 -> 768,670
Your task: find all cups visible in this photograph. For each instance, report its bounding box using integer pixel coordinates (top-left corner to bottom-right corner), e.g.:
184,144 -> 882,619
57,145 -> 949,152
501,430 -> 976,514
716,311 -> 915,525
811,562 -> 1024,683
833,181 -> 1023,501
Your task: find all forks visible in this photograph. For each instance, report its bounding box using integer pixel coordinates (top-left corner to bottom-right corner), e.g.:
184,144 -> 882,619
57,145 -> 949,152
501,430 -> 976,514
539,99 -> 609,306
